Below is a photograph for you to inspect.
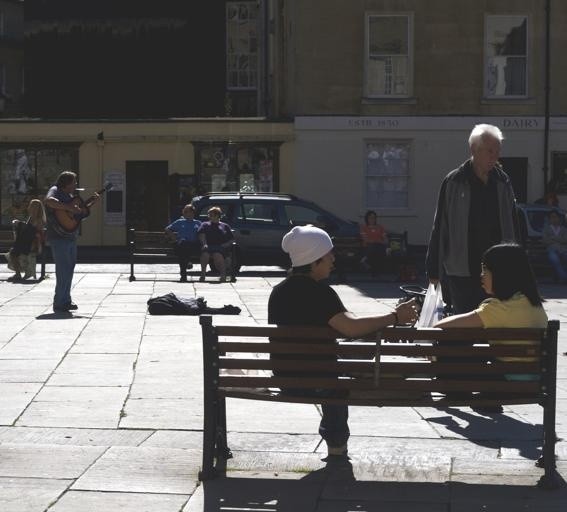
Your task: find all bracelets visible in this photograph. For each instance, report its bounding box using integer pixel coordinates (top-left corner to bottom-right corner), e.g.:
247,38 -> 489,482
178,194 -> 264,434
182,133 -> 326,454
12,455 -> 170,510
389,309 -> 398,328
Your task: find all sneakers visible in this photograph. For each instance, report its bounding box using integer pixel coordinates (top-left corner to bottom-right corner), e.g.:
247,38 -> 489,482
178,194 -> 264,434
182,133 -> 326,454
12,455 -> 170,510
7,274 -> 31,282
437,394 -> 502,413
54,302 -> 77,317
318,420 -> 348,456
186,261 -> 193,269
180,274 -> 227,282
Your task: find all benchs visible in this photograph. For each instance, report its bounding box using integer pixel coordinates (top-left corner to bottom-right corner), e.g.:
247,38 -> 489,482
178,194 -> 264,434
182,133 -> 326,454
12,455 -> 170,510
198,314 -> 565,490
128,228 -> 237,282
331,231 -> 413,285
0,228 -> 47,279
525,236 -> 555,284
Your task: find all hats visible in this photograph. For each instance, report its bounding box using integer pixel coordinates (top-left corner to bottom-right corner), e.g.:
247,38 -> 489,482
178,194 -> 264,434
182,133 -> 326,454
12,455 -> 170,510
281,225 -> 334,267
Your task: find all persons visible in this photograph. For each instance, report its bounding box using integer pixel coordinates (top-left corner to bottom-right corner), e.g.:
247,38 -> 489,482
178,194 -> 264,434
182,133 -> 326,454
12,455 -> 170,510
2,198 -> 46,284
530,188 -> 559,226
356,209 -> 395,272
195,205 -> 233,280
426,238 -> 549,413
423,124 -> 524,317
41,170 -> 100,316
265,225 -> 419,459
164,203 -> 203,281
542,208 -> 566,282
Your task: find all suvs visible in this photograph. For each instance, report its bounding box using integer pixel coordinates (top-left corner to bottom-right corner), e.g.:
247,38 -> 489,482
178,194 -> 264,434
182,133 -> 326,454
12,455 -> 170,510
180,190 -> 376,276
518,202 -> 567,272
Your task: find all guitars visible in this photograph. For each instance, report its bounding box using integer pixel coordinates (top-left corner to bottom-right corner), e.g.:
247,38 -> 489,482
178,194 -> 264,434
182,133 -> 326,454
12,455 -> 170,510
53,182 -> 114,233
